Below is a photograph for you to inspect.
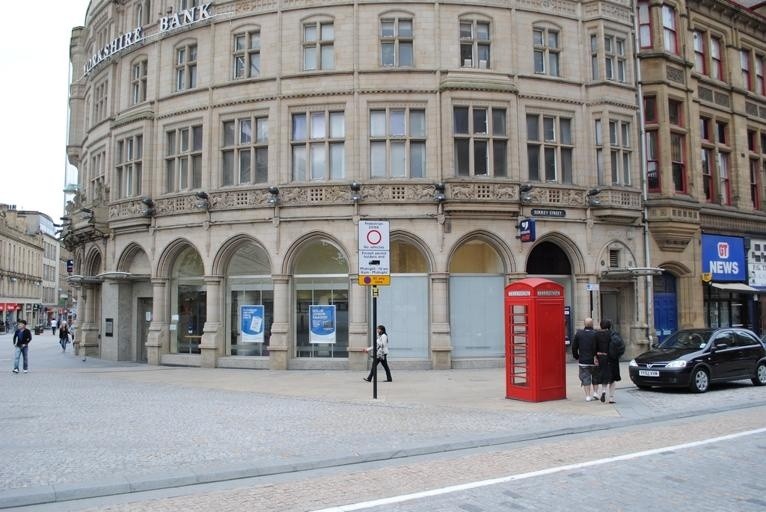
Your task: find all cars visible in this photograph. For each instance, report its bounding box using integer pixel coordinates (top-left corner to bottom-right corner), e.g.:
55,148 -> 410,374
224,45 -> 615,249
629,327 -> 766,394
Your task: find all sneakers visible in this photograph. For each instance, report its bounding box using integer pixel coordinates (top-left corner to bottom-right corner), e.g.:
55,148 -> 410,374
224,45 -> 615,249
384,378 -> 392,382
585,391 -> 616,404
12,368 -> 28,373
363,377 -> 371,382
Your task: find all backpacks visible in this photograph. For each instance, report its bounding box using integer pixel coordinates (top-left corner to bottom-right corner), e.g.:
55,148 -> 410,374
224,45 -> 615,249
608,329 -> 625,361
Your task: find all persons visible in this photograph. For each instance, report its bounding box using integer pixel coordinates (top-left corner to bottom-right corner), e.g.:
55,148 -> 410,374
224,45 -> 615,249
572,317 -> 625,403
12,319 -> 32,373
51,317 -> 57,336
5,318 -> 11,333
59,320 -> 75,351
362,325 -> 393,382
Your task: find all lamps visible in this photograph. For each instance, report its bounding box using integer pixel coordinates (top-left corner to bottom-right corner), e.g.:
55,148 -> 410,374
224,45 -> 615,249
432,181 -> 445,201
195,190 -> 211,211
141,198 -> 156,217
349,181 -> 360,202
80,207 -> 95,224
59,216 -> 72,230
267,185 -> 281,205
584,187 -> 601,207
519,183 -> 533,207
